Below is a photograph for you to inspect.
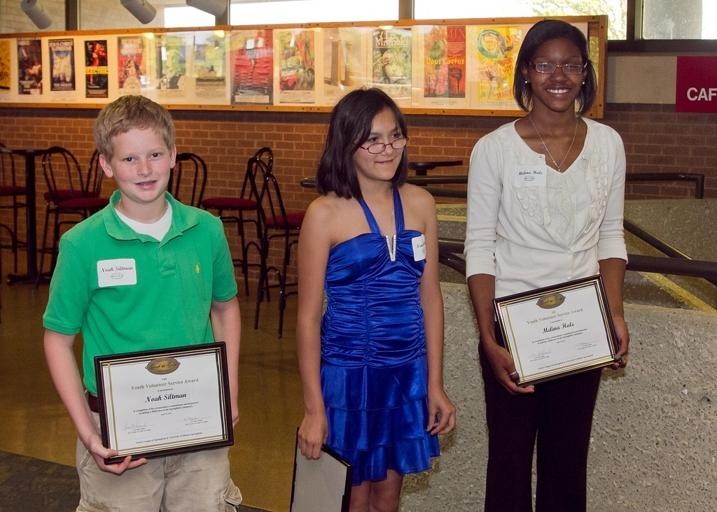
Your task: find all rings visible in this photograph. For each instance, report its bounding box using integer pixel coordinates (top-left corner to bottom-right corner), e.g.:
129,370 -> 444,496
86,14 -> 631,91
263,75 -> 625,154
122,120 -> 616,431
508,370 -> 516,376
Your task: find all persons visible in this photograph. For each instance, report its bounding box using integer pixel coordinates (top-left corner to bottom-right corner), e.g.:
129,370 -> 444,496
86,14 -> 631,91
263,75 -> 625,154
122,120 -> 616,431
40,96 -> 243,512
463,20 -> 628,511
294,88 -> 457,511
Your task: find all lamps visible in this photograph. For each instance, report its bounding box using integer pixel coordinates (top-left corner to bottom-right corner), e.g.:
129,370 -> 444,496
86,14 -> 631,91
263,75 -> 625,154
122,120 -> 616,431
19,0 -> 228,28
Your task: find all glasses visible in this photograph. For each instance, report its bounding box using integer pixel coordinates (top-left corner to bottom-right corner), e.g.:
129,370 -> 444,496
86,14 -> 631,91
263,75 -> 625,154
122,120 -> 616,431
525,59 -> 589,74
358,137 -> 409,154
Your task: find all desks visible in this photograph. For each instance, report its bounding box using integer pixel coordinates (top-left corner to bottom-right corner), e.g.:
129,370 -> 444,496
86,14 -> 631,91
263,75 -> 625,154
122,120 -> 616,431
13,144 -> 54,281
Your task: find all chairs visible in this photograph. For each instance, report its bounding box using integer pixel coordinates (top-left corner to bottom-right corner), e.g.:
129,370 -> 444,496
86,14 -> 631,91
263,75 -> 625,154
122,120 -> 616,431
200,148 -> 276,303
244,158 -> 308,339
39,149 -> 102,273
4,147 -> 28,284
34,144 -> 105,286
163,153 -> 209,210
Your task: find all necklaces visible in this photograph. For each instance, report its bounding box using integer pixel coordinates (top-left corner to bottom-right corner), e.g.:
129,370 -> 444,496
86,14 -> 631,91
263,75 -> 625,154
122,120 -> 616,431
530,110 -> 582,172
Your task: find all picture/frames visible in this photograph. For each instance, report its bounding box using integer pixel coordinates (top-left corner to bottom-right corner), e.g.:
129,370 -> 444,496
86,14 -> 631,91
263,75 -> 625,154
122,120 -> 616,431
94,342 -> 235,466
0,16 -> 607,120
490,274 -> 623,388
